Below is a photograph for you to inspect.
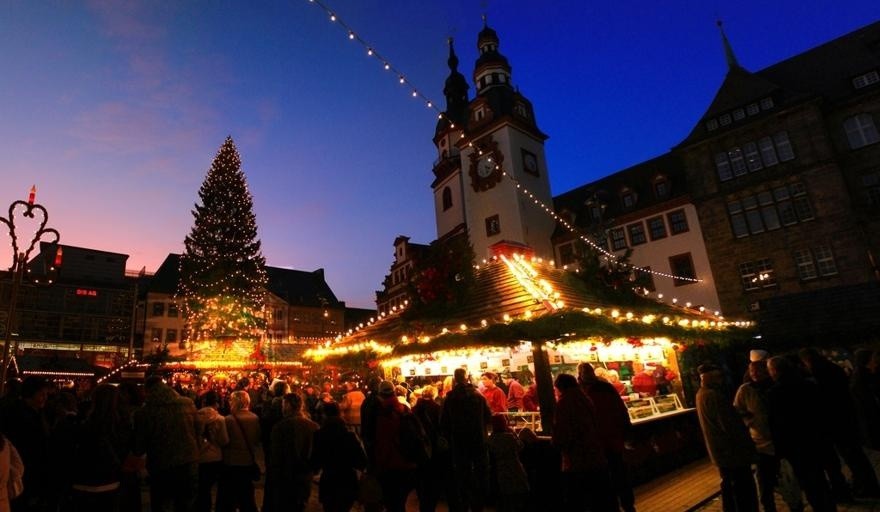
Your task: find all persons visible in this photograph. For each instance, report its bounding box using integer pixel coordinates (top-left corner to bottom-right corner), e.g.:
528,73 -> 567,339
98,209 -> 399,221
694,348 -> 880,512
0,362 -> 636,512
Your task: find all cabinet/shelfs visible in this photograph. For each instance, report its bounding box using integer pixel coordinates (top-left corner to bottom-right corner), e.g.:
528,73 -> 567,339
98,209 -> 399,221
624,392 -> 706,486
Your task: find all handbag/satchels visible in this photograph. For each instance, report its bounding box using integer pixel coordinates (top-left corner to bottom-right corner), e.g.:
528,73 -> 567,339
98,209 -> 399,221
251,461 -> 262,482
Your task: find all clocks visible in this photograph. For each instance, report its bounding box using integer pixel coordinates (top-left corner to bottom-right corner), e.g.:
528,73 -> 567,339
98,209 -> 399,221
467,135 -> 504,192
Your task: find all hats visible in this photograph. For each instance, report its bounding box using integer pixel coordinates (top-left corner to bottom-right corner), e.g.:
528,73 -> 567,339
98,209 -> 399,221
750,350 -> 770,363
379,382 -> 393,395
696,364 -> 725,375
